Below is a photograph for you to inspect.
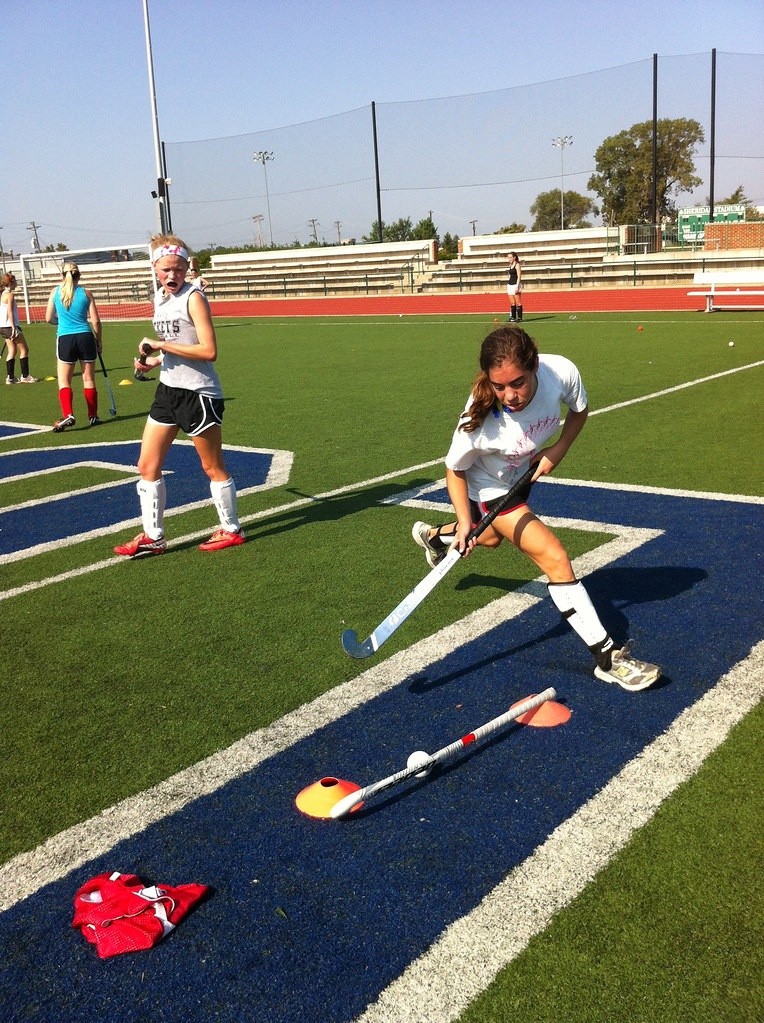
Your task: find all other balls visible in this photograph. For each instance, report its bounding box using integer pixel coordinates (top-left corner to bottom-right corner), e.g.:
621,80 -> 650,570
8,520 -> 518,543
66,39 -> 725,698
407,751 -> 433,778
728,342 -> 734,346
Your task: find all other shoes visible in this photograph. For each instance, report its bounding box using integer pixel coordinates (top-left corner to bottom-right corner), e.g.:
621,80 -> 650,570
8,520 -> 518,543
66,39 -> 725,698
518,318 -> 523,322
510,318 -> 516,322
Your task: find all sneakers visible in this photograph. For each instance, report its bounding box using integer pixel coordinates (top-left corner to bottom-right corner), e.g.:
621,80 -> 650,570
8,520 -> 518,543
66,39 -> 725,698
114,532 -> 166,556
6,375 -> 21,385
53,413 -> 75,432
198,527 -> 246,551
89,416 -> 102,426
412,519 -> 448,569
20,374 -> 39,383
593,637 -> 663,692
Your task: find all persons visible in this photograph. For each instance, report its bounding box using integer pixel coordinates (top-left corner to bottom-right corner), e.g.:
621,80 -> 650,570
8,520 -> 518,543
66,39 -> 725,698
46,261 -> 103,432
190,268 -> 209,293
0,273 -> 38,384
507,251 -> 523,321
412,324 -> 661,691
114,235 -> 246,555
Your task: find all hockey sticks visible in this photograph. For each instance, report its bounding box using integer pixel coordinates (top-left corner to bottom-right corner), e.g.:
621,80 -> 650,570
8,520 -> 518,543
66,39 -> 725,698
340,461 -> 541,661
0,342 -> 6,359
133,343 -> 156,382
328,686 -> 558,818
98,350 -> 118,416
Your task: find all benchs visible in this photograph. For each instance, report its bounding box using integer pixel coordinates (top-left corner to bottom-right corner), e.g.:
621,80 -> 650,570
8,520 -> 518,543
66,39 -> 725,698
687,272 -> 764,313
11,241 -> 619,305
